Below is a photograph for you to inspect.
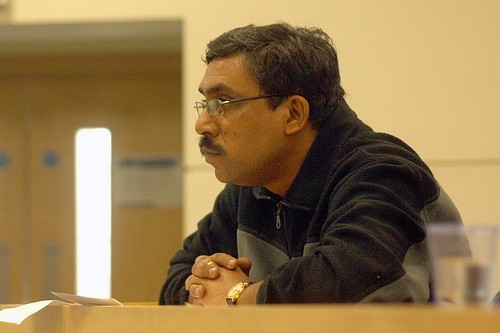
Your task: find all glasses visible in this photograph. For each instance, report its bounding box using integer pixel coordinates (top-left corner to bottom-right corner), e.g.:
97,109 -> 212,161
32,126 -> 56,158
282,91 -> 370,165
196,91 -> 304,120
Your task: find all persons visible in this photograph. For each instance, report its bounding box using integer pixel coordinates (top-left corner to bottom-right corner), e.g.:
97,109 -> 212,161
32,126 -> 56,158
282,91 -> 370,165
159,22 -> 474,308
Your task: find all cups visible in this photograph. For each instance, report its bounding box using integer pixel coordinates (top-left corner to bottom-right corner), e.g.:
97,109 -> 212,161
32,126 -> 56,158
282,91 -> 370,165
424,224 -> 500,310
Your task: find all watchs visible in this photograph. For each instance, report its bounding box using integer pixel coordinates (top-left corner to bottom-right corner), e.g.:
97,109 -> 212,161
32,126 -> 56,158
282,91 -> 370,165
225,282 -> 256,306
